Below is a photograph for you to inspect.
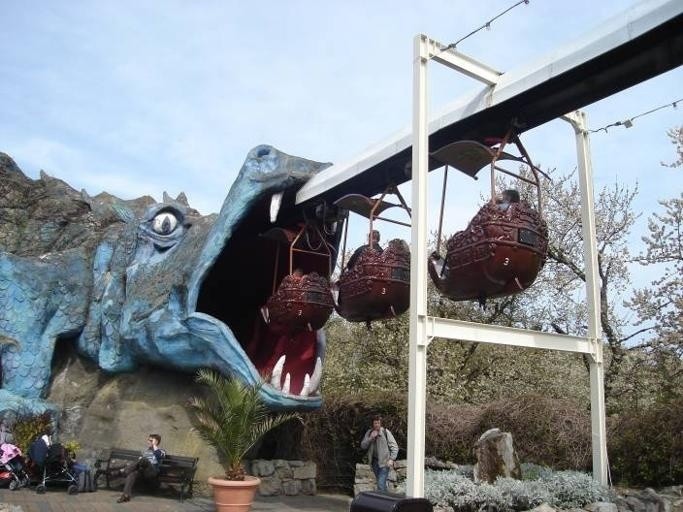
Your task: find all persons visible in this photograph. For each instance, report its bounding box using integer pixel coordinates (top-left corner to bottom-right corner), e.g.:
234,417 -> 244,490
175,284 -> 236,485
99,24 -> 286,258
346,229 -> 383,271
495,190 -> 521,212
291,266 -> 302,278
358,415 -> 399,492
108,432 -> 166,503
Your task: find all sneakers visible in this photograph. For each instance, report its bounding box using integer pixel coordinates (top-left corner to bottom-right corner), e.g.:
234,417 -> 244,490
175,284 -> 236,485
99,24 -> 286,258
115,496 -> 130,502
106,470 -> 120,479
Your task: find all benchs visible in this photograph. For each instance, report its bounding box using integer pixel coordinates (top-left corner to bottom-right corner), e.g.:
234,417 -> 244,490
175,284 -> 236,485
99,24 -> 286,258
93,450 -> 199,503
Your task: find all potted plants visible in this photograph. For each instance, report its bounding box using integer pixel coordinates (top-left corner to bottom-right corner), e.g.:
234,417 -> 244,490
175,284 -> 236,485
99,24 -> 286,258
184,368 -> 304,512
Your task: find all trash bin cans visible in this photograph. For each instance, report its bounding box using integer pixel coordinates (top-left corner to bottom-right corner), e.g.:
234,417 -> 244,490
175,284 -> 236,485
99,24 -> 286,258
350,491 -> 433,512
